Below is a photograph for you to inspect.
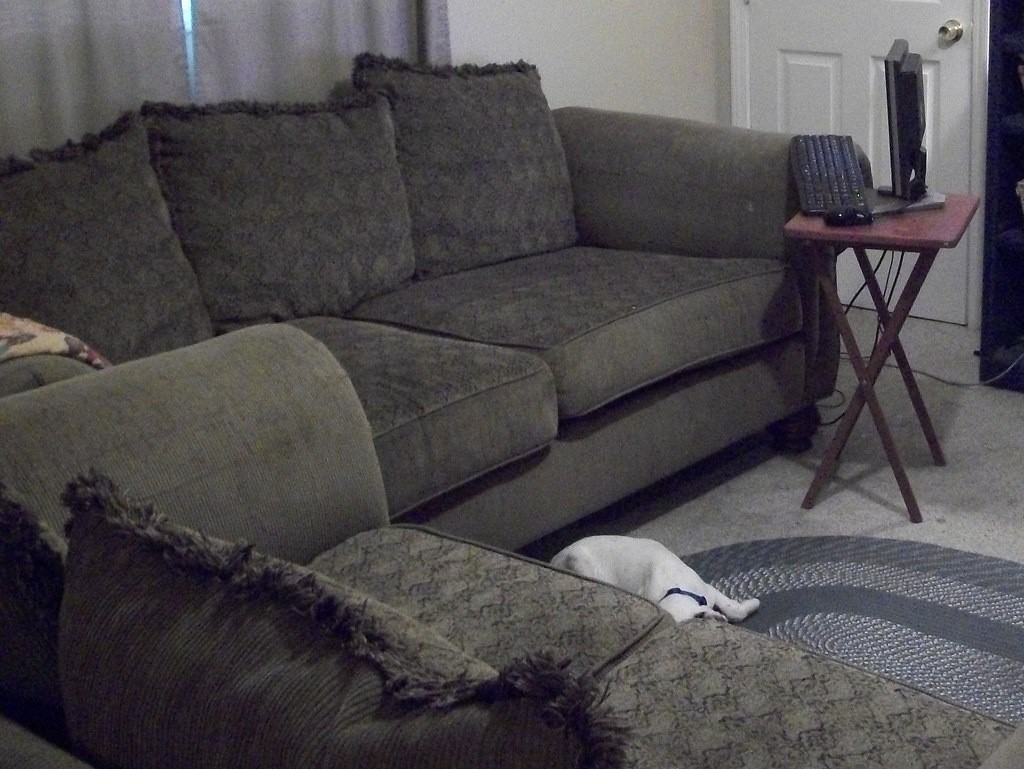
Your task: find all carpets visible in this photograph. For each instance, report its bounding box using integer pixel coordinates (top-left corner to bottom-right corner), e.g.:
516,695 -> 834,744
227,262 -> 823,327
676,533 -> 1024,731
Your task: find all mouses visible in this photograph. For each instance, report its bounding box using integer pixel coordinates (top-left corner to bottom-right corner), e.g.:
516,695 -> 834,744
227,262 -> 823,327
820,206 -> 874,226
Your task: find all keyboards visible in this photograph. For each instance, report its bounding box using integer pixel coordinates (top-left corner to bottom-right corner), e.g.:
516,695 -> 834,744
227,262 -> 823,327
789,133 -> 873,214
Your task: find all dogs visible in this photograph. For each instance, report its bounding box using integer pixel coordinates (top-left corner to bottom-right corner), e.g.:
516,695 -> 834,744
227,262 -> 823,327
549,534 -> 760,623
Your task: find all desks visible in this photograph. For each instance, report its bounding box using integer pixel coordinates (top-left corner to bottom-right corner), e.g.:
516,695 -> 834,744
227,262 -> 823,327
785,189 -> 981,525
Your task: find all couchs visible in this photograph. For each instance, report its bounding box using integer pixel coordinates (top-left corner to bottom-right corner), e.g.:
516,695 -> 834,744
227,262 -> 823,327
0,104 -> 1024,769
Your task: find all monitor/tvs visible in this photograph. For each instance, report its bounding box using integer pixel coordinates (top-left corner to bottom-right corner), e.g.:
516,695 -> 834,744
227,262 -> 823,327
874,38 -> 927,217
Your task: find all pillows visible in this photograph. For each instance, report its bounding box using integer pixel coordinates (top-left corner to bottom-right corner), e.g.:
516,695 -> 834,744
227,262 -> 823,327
145,95 -> 419,333
1,486 -> 71,754
57,470 -> 623,768
349,50 -> 582,281
2,111 -> 215,371
